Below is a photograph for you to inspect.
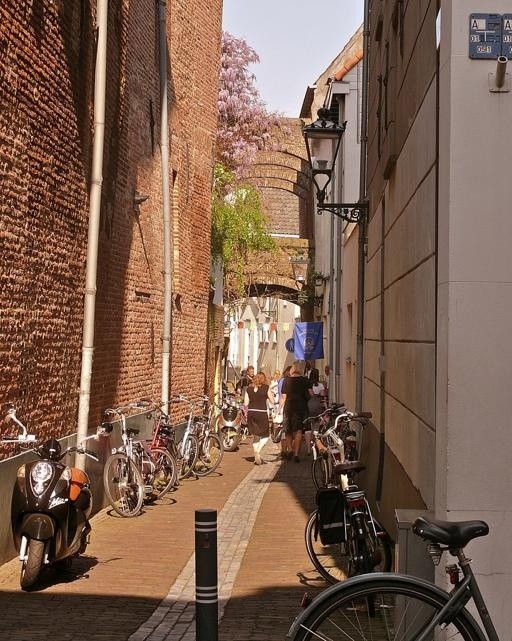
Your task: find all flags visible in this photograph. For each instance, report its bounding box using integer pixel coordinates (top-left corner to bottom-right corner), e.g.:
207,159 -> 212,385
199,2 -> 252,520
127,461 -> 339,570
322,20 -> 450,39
263,323 -> 269,330
244,321 -> 250,328
277,322 -> 283,330
270,322 -> 277,331
250,322 -> 257,329
283,322 -> 290,330
293,320 -> 325,360
257,322 -> 263,329
238,321 -> 244,328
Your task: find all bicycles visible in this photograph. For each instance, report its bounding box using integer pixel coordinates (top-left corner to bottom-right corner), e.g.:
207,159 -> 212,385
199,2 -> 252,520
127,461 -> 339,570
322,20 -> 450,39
304,410 -> 397,618
302,399 -> 360,493
102,393 -> 223,518
287,515 -> 502,641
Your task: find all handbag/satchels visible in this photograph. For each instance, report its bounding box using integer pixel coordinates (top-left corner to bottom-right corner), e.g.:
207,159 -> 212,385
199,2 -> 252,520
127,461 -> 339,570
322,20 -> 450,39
313,486 -> 347,547
271,421 -> 283,443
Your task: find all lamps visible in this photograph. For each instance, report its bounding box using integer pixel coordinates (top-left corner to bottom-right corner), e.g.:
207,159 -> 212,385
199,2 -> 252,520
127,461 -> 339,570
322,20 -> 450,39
301,103 -> 370,256
257,296 -> 278,322
289,247 -> 323,312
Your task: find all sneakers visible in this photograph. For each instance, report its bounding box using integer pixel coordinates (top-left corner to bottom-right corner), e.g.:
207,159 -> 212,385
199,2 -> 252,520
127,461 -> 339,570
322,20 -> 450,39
294,453 -> 300,462
254,450 -> 261,464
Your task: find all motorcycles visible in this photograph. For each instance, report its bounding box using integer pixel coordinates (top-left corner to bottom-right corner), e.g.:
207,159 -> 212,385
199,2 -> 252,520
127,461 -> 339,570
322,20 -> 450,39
217,396 -> 247,452
0,408 -> 113,591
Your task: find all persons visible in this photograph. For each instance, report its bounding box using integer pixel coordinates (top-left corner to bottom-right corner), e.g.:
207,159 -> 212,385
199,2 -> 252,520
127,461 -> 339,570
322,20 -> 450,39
235,359 -> 330,465
